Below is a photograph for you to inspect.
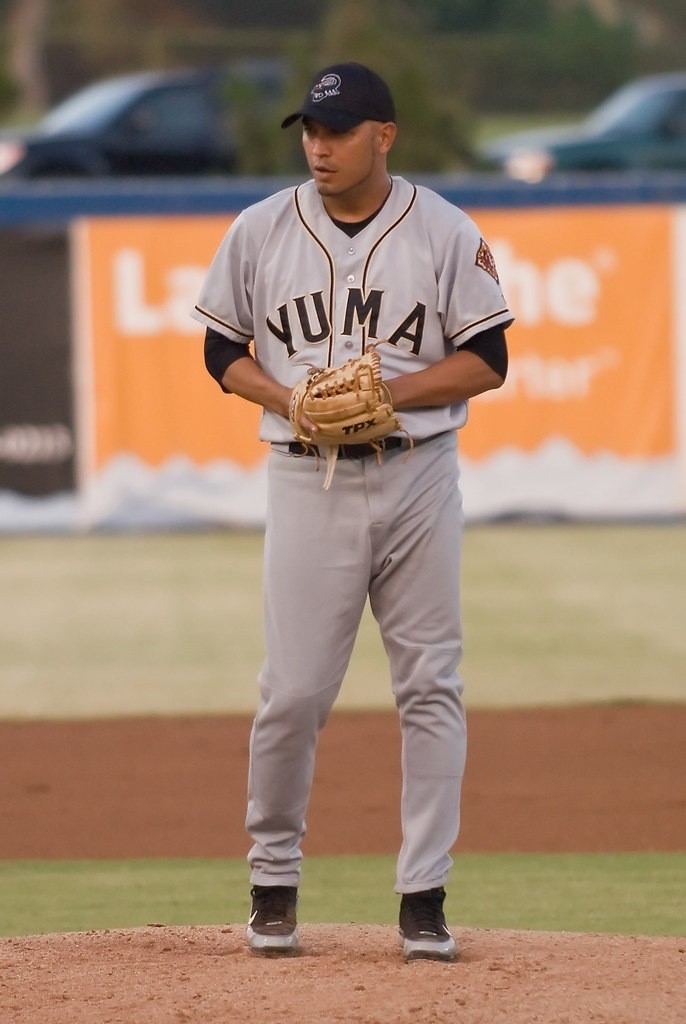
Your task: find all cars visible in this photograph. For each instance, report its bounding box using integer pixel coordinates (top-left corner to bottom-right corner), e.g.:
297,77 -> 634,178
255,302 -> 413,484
0,66 -> 303,184
469,67 -> 686,178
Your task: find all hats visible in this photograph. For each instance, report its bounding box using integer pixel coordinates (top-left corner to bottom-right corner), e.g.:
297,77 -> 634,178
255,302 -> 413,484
281,62 -> 397,131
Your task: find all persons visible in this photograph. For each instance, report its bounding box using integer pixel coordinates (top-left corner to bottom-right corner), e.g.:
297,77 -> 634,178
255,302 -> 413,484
190,63 -> 514,960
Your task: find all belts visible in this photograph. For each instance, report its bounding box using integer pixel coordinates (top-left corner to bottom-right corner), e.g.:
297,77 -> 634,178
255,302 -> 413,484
289,437 -> 402,458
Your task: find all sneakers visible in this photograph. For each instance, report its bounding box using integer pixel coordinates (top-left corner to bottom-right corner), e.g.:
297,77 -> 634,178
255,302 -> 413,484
396,886 -> 456,961
246,884 -> 299,950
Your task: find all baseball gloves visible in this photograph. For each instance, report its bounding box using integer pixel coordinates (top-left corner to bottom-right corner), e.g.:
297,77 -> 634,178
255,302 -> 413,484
287,351 -> 400,448
